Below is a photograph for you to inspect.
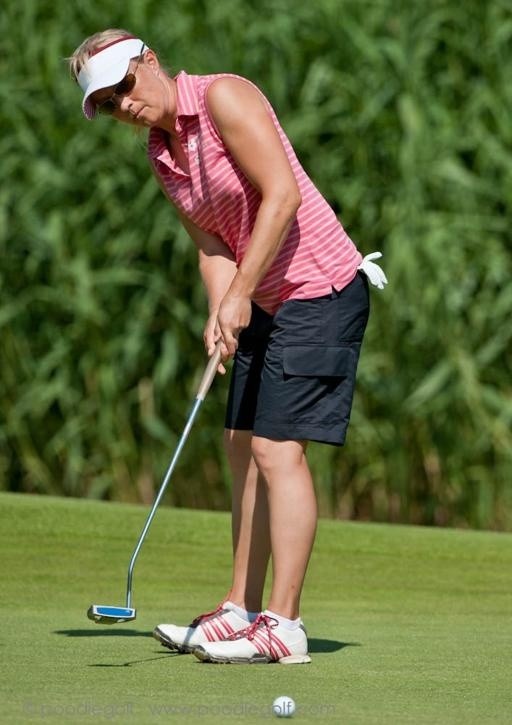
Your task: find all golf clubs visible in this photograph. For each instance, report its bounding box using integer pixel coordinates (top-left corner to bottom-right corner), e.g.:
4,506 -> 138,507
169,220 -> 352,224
87,335 -> 224,624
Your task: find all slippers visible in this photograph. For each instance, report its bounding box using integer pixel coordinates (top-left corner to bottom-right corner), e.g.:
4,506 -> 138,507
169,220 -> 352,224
98,43 -> 146,115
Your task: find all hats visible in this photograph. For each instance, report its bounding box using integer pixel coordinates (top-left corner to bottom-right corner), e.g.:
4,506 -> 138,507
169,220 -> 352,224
76,35 -> 151,121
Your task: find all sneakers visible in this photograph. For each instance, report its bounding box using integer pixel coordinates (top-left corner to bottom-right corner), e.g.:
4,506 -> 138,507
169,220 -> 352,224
153,608 -> 312,664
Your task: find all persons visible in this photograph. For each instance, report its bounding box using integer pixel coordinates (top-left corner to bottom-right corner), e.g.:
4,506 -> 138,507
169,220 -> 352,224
59,25 -> 390,666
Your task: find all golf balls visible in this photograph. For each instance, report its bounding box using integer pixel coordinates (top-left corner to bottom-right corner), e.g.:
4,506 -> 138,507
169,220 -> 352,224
272,695 -> 296,718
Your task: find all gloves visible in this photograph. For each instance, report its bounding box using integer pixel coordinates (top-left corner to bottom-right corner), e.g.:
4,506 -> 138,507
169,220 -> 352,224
357,251 -> 388,290
